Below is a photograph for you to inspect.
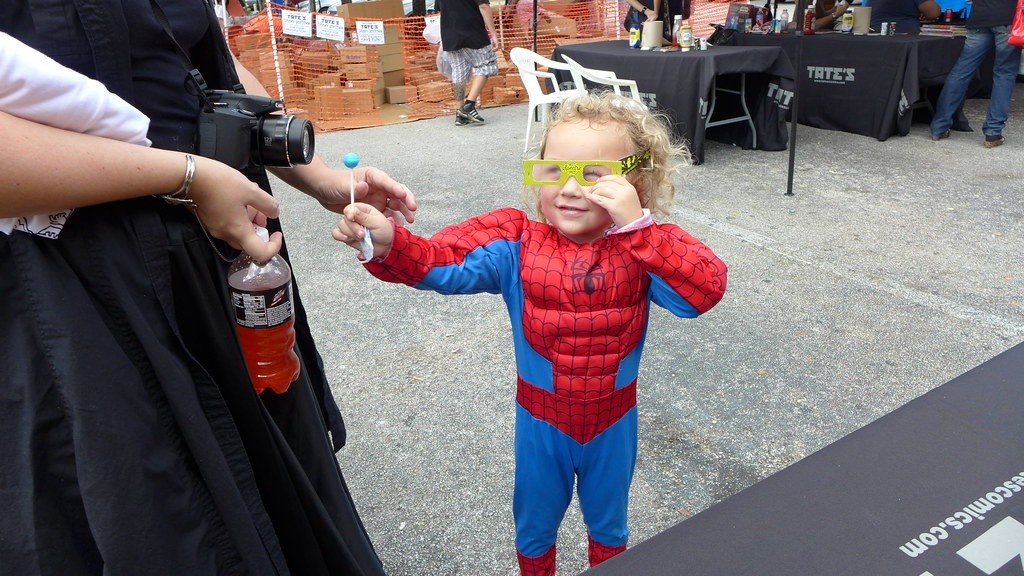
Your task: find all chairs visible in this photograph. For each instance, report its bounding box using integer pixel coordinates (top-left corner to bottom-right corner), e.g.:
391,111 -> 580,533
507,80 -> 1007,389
560,53 -> 641,102
509,47 -> 589,153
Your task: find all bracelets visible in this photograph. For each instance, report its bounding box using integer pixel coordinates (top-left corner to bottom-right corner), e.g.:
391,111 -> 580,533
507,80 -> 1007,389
642,6 -> 647,13
152,155 -> 196,207
832,13 -> 838,20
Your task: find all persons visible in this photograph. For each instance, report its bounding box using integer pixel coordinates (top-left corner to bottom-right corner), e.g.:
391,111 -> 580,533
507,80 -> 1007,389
332,92 -> 728,576
0,0 -> 418,576
927,0 -> 1022,148
433,0 -> 499,126
624,0 -> 690,40
794,0 -> 849,31
871,0 -> 941,33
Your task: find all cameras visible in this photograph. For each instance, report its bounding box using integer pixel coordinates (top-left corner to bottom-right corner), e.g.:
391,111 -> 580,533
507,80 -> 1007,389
197,83 -> 315,170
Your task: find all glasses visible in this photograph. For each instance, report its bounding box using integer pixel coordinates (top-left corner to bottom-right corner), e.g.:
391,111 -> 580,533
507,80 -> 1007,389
521,148 -> 655,189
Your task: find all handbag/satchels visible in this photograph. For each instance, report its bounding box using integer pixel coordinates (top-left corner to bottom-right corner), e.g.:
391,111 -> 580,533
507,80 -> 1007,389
624,5 -> 638,32
1007,0 -> 1024,49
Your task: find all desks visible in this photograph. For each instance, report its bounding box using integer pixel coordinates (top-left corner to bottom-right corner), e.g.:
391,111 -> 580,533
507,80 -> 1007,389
553,40 -> 793,165
707,28 -> 974,143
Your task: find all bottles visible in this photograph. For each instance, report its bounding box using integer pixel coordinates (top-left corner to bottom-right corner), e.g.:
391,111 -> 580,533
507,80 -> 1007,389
672,15 -> 683,48
775,21 -> 781,36
842,10 -> 854,33
701,38 -> 707,51
881,23 -> 888,35
770,17 -> 776,34
228,226 -> 299,395
780,9 -> 789,33
694,37 -> 700,51
680,26 -> 692,52
889,22 -> 897,36
629,10 -> 641,49
804,5 -> 816,34
756,8 -> 763,29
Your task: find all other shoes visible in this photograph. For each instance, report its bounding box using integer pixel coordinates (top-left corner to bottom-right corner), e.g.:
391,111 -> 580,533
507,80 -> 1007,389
931,128 -> 951,139
983,134 -> 1005,149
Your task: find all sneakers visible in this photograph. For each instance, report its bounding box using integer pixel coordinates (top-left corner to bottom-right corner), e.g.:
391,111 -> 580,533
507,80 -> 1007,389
457,102 -> 485,124
455,111 -> 474,127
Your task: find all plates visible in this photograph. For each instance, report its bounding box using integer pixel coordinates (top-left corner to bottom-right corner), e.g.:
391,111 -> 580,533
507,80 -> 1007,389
750,31 -> 763,34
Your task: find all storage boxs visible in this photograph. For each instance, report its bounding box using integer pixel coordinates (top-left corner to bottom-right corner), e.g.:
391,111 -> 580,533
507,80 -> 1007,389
226,1 -> 610,113
